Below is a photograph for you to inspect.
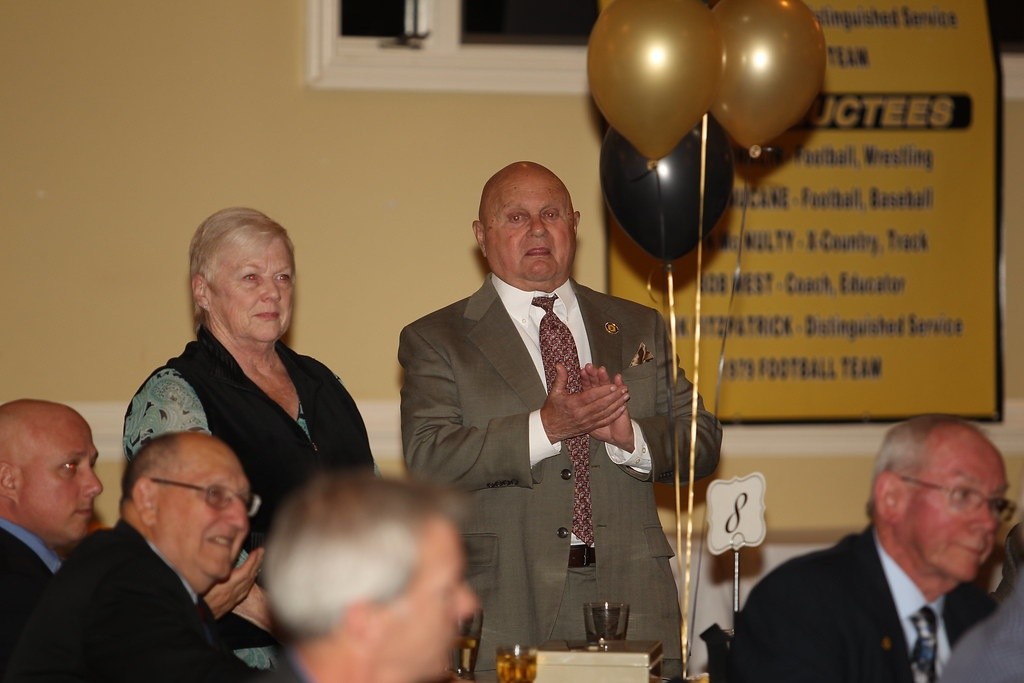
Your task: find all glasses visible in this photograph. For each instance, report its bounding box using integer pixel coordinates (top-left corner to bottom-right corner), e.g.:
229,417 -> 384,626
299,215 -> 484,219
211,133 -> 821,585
900,475 -> 1017,524
149,476 -> 263,519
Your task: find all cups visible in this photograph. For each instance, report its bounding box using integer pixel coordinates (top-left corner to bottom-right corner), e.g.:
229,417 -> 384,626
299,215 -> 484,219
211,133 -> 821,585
583,603 -> 628,642
448,608 -> 484,680
495,645 -> 538,683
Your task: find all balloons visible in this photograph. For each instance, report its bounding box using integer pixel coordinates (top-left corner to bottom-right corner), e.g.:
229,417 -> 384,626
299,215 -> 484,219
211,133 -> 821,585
711,0 -> 827,158
600,114 -> 733,270
587,0 -> 713,169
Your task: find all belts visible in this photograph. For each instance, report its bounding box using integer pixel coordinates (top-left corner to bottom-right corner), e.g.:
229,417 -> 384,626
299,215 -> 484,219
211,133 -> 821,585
568,545 -> 598,567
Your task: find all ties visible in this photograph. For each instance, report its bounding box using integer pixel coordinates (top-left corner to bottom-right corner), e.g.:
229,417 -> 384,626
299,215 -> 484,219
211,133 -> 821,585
530,293 -> 595,550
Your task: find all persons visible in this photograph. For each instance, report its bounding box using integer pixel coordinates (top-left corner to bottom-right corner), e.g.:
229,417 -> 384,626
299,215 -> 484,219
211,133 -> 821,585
397,162 -> 722,677
0,400 -> 263,652
0,433 -> 263,683
239,476 -> 477,683
723,416 -> 1024,683
122,208 -> 376,671
